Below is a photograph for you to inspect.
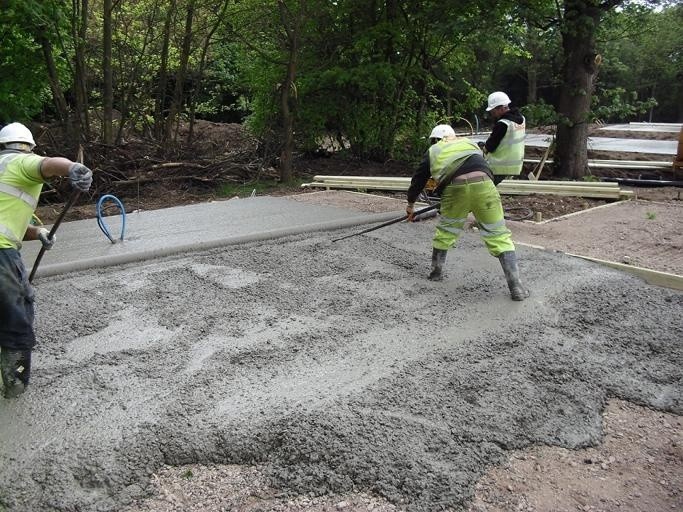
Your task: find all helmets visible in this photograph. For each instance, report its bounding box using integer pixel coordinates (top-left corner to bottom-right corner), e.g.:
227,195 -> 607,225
429,124 -> 456,140
0,122 -> 35,150
483,92 -> 512,112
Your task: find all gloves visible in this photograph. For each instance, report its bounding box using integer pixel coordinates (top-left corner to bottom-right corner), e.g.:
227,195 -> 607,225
68,162 -> 92,193
37,227 -> 55,251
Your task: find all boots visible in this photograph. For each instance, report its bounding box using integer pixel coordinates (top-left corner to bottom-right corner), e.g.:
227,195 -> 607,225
0,348 -> 31,398
428,248 -> 447,282
495,251 -> 530,300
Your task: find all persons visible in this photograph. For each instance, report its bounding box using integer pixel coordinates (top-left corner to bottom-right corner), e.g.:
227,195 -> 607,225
0,122 -> 94,397
404,123 -> 531,302
478,92 -> 526,186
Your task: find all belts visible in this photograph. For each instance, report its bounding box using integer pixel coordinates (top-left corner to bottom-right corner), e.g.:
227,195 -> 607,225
448,174 -> 490,186
